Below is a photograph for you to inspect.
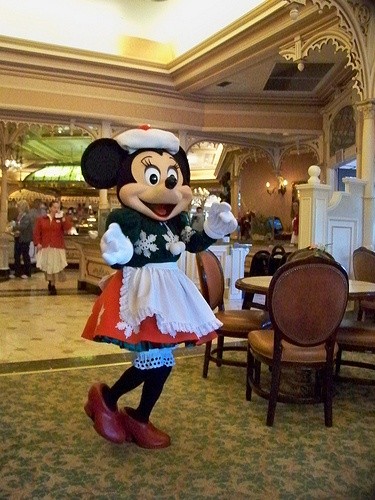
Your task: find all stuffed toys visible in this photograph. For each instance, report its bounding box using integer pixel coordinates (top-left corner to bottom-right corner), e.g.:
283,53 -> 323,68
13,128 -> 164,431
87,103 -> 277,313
81,127 -> 239,450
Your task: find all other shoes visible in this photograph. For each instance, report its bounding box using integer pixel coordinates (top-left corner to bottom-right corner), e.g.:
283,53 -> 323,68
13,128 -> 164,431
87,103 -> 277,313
48,284 -> 57,294
21,275 -> 30,278
9,274 -> 19,278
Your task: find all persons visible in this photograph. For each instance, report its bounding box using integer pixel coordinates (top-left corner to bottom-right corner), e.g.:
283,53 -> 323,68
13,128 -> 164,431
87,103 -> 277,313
191,207 -> 205,224
5,200 -> 32,279
34,200 -> 73,295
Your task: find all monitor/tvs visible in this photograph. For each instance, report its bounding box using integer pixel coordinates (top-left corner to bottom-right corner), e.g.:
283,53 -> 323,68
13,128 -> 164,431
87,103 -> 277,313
268,217 -> 283,231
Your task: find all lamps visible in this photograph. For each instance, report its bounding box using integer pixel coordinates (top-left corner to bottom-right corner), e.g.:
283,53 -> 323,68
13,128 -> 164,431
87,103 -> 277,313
265,175 -> 287,197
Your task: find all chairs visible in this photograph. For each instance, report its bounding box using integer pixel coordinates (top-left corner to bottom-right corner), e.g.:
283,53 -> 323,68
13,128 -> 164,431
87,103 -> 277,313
196,249 -> 267,402
286,247 -> 375,322
245,257 -> 349,428
336,326 -> 375,391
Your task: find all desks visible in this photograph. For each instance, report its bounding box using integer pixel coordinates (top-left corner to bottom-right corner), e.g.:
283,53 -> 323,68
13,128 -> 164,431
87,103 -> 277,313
235,274 -> 375,385
